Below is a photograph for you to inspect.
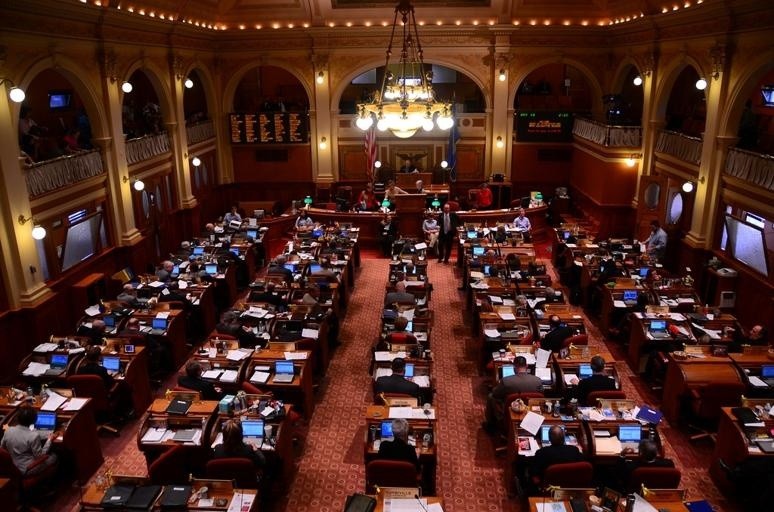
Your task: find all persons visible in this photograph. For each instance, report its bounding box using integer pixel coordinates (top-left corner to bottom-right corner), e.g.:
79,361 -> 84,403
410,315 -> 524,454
735,99 -> 758,150
460,209 -> 774,496
2,180 -> 459,490
19,96 -> 159,162
256,94 -> 290,112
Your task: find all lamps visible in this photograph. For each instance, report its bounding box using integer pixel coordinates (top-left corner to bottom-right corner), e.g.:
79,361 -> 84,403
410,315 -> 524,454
496,135 -> 505,148
122,175 -> 145,192
373,159 -> 382,170
353,0 -> 457,141
624,151 -> 644,169
0,74 -> 26,106
109,72 -> 133,94
17,214 -> 46,242
682,176 -> 706,194
177,73 -> 193,90
498,68 -> 506,83
441,160 -> 452,171
634,72 -> 651,87
695,73 -> 719,90
319,137 -> 326,150
184,152 -> 202,168
315,71 -> 326,85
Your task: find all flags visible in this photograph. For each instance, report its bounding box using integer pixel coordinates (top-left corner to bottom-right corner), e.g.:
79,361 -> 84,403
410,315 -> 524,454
364,126 -> 378,193
447,100 -> 461,183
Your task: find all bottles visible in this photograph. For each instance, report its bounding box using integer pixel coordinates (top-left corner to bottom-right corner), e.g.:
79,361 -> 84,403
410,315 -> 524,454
234,397 -> 240,411
554,401 -> 560,417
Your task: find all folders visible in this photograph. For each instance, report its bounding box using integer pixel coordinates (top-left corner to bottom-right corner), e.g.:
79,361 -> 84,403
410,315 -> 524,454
636,405 -> 663,425
686,501 -> 713,512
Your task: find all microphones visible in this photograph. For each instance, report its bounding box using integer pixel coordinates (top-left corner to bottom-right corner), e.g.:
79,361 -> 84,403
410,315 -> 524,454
413,490 -> 431,511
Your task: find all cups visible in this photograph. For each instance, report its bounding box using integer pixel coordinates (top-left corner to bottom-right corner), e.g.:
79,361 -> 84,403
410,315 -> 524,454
25,396 -> 37,406
114,344 -> 122,351
499,349 -> 506,358
264,426 -> 272,439
369,427 -> 376,441
425,350 -> 431,359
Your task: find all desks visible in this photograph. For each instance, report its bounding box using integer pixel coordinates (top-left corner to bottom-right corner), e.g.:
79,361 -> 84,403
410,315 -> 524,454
0,219 -> 773,511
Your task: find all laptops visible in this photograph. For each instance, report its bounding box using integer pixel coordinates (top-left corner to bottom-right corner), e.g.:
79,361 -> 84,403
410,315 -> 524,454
32,212 -> 258,442
377,239 -> 419,444
459,225 -> 645,460
559,228 -> 774,398
238,225 -> 349,451
